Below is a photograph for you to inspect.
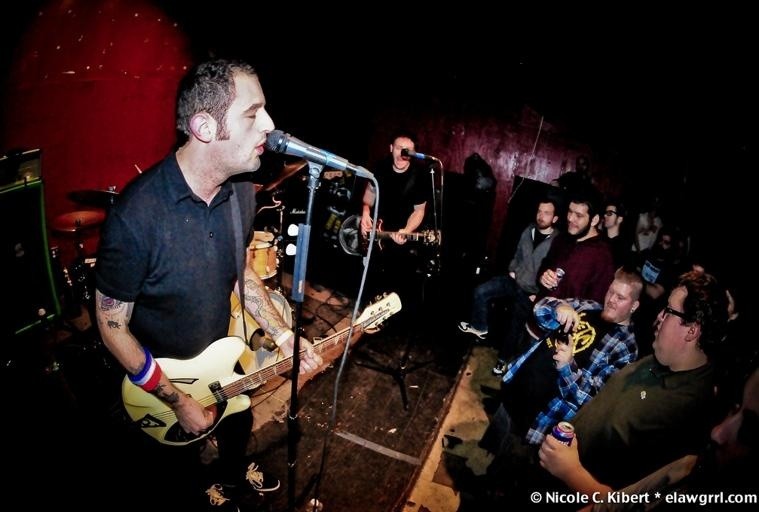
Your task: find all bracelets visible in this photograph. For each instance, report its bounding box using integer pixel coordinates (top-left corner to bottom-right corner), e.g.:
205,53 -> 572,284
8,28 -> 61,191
275,329 -> 294,347
125,346 -> 161,393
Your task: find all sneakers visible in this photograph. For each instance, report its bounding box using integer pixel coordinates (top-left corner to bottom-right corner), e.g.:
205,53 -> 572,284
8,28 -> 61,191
458,321 -> 489,340
492,359 -> 506,374
222,470 -> 281,491
202,486 -> 240,511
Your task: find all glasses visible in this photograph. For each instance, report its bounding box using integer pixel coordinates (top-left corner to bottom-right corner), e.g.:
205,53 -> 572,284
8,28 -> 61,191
663,307 -> 695,324
603,210 -> 616,216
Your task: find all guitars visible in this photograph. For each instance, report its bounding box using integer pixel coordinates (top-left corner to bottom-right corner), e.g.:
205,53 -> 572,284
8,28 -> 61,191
123,293 -> 403,445
338,215 -> 441,255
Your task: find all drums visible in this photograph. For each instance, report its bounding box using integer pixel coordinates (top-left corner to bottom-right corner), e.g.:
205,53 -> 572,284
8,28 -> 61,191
247,231 -> 278,280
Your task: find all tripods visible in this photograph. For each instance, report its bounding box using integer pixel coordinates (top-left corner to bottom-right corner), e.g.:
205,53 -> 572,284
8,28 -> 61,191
355,254 -> 437,411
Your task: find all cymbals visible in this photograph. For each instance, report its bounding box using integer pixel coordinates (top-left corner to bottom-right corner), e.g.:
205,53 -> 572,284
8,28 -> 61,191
52,208 -> 105,231
65,189 -> 120,203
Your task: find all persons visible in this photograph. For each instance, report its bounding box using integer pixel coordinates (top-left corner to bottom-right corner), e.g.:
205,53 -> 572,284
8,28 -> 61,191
482,184 -> 758,512
359,133 -> 427,330
92,55 -> 323,512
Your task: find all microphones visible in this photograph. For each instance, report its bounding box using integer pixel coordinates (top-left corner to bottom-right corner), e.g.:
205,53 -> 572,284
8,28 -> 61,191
264,129 -> 366,177
401,148 -> 435,163
